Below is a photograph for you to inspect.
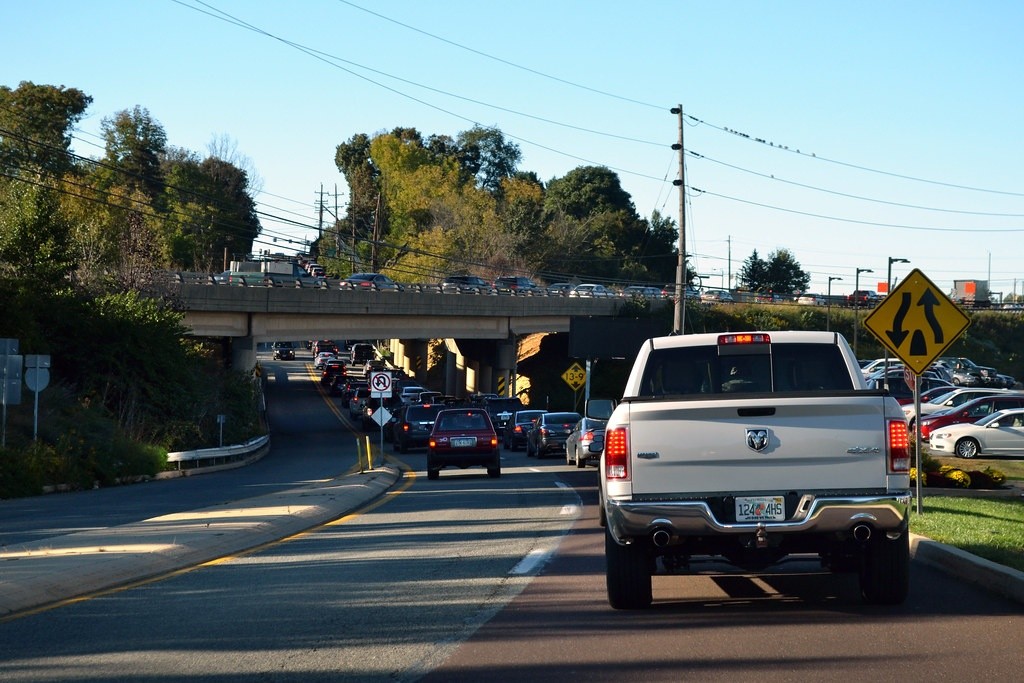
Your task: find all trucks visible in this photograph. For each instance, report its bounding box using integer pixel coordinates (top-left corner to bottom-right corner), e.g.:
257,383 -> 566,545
953,279 -> 992,307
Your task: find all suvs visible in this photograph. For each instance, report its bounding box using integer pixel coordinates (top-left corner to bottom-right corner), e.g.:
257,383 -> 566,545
491,276 -> 544,296
442,275 -> 493,295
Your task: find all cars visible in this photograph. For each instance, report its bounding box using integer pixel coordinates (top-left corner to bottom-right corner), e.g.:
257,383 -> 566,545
307,340 -> 608,481
298,259 -> 327,280
858,356 -> 1024,459
797,293 -> 827,307
546,282 -> 614,300
270,341 -> 296,360
1002,303 -> 1021,309
620,283 -> 735,304
337,273 -> 405,293
213,270 -> 231,285
266,372 -> 278,385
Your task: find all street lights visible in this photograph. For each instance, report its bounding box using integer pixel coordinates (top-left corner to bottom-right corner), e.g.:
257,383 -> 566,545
827,276 -> 844,332
884,257 -> 910,389
854,268 -> 874,358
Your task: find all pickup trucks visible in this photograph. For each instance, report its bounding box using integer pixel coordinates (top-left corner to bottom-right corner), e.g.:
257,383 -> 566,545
847,290 -> 877,307
583,330 -> 913,610
229,261 -> 321,290
